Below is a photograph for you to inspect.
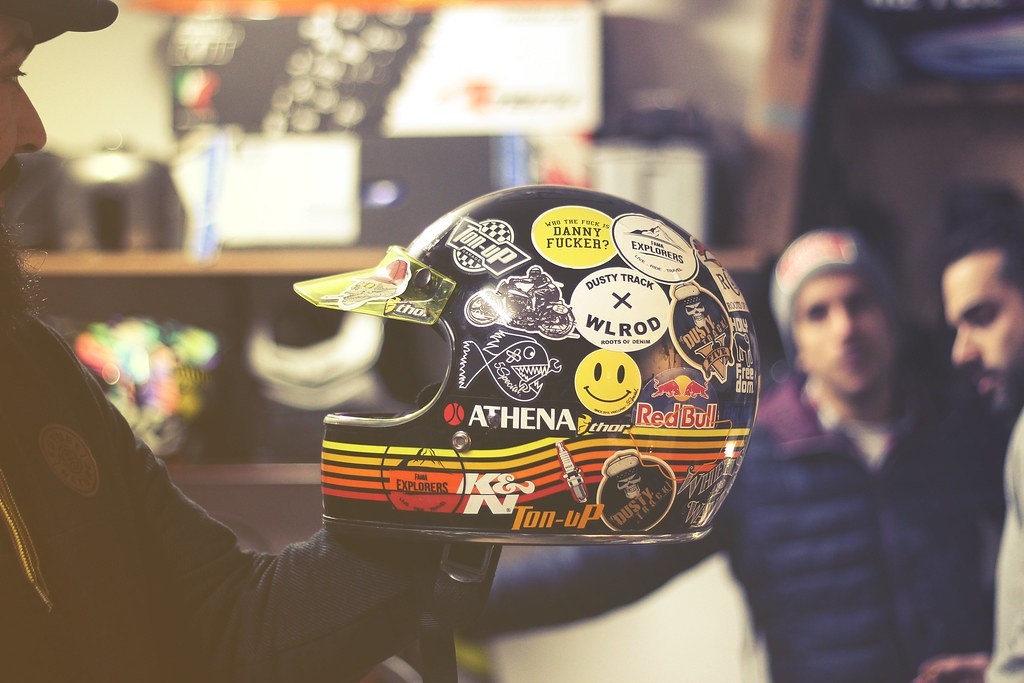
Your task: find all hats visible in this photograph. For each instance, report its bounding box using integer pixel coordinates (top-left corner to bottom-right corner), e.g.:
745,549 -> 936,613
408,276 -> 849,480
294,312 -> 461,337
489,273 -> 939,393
0,0 -> 118,45
772,227 -> 898,359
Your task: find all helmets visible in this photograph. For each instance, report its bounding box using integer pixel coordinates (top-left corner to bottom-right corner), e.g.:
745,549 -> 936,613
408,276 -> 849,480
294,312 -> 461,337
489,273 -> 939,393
293,184 -> 761,546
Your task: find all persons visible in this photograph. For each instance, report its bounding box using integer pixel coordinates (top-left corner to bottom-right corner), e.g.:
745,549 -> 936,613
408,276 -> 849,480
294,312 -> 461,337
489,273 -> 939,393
0,0 -> 489,683
453,175 -> 1024,683
